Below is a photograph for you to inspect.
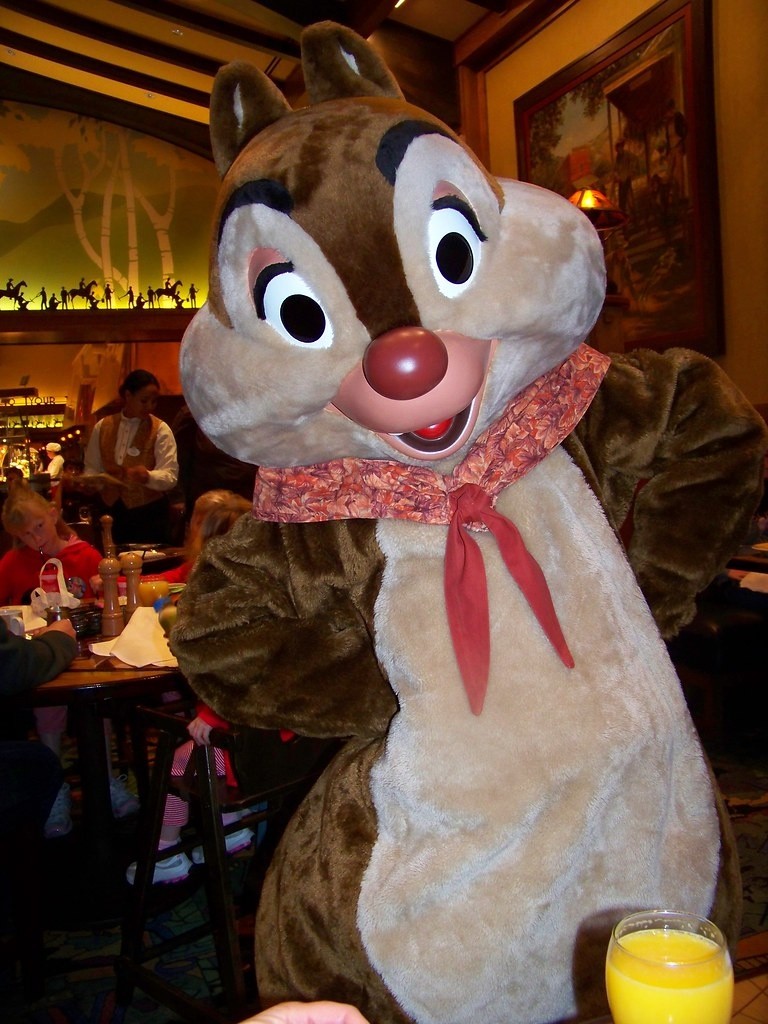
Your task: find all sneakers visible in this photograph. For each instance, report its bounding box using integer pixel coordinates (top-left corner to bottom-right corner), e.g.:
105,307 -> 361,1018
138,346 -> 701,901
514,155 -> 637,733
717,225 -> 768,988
192,825 -> 256,865
111,778 -> 140,816
46,783 -> 74,837
127,851 -> 193,885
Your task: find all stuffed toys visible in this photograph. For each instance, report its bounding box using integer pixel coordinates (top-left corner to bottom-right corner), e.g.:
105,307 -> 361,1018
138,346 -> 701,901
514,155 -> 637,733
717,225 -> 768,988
167,20 -> 768,1024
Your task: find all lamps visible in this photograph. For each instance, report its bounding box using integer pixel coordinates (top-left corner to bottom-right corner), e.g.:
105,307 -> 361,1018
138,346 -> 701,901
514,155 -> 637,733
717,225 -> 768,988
565,187 -> 628,314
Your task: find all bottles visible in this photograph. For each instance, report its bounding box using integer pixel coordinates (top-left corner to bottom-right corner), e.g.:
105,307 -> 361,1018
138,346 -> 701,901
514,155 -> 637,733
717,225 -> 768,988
159,600 -> 177,634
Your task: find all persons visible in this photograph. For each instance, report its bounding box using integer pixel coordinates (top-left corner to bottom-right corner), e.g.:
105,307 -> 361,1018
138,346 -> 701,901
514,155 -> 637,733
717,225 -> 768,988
236,1000 -> 371,1024
5,277 -> 200,311
0,369 -> 256,887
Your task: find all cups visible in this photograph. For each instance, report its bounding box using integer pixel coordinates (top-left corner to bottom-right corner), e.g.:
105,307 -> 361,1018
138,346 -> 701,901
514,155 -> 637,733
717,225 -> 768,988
138,579 -> 168,607
43,606 -> 71,627
0,610 -> 25,638
605,910 -> 734,1024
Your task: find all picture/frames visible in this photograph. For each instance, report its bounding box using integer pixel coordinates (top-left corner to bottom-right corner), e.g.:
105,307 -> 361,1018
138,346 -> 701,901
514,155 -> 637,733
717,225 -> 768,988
514,0 -> 723,364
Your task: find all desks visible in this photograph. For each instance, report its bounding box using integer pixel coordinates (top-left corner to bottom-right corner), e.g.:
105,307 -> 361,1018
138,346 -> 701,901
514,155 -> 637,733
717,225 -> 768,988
12,543 -> 282,1021
